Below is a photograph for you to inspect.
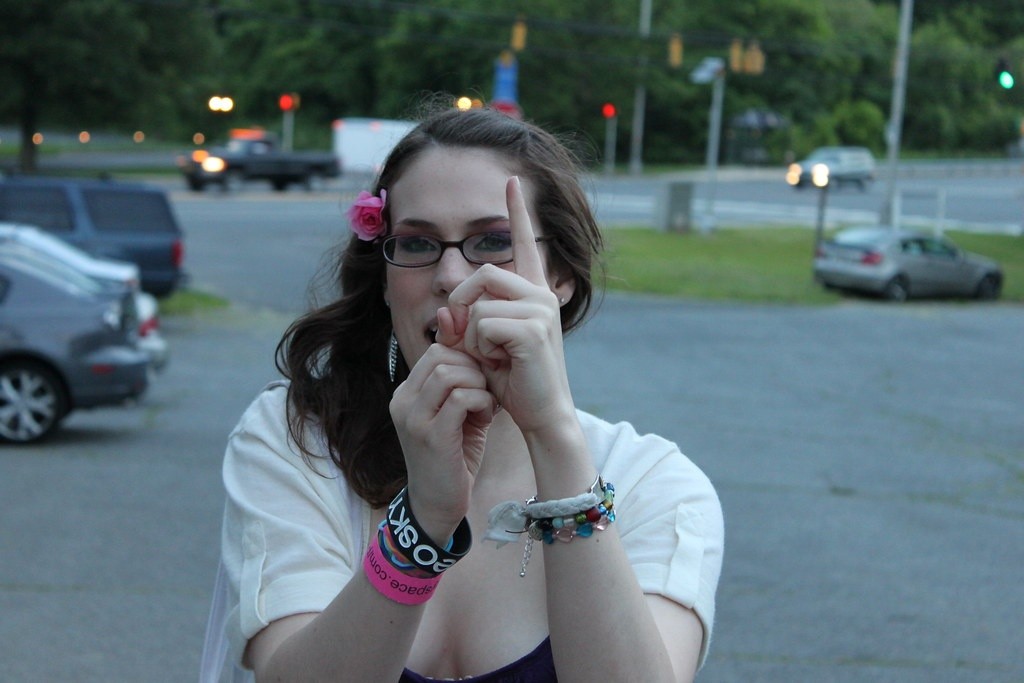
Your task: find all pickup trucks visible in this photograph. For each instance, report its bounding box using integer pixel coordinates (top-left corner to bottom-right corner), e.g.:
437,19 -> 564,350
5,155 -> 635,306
184,132 -> 342,194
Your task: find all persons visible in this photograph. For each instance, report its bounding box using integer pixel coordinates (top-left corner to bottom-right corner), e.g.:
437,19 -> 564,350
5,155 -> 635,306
195,108 -> 729,676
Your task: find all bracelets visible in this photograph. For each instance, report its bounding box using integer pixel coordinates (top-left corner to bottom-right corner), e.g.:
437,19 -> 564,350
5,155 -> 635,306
483,473 -> 619,580
361,486 -> 475,610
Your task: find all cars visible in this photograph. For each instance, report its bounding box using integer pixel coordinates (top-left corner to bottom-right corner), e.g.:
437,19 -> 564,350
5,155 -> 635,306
1,223 -> 171,446
814,222 -> 1007,306
1,172 -> 185,301
788,145 -> 877,192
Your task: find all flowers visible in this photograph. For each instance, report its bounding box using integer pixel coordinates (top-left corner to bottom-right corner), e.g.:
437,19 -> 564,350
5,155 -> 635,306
340,185 -> 389,244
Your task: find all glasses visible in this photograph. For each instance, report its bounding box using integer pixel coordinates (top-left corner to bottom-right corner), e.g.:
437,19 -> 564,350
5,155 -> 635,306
381,230 -> 557,268
